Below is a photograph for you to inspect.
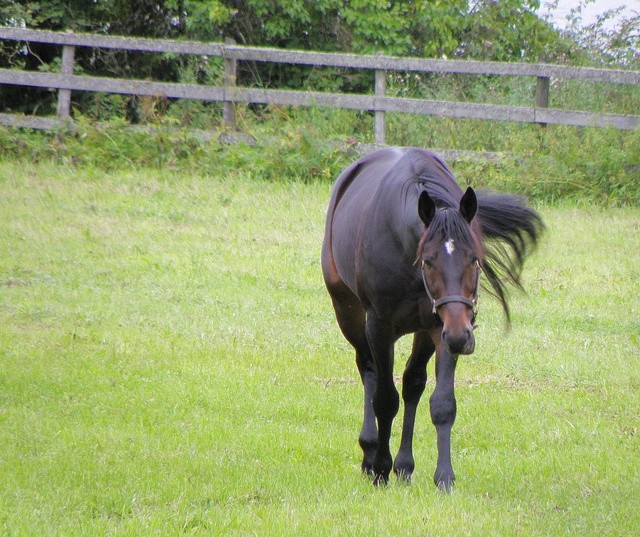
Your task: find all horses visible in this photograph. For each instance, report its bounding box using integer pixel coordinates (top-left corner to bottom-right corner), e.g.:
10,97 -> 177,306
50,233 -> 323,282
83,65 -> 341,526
320,146 -> 547,495
